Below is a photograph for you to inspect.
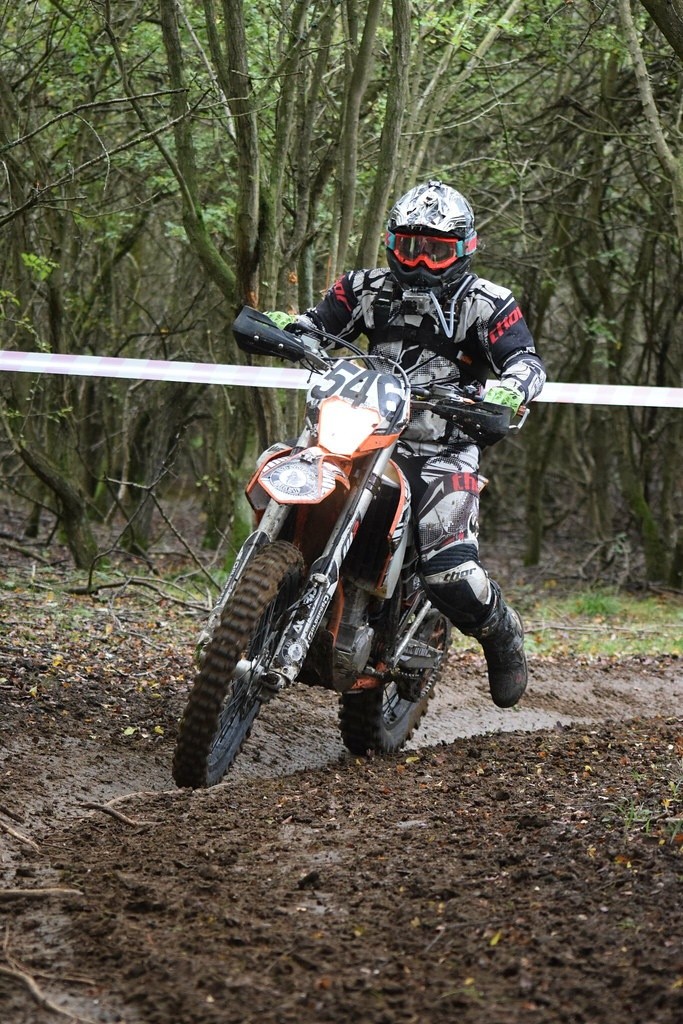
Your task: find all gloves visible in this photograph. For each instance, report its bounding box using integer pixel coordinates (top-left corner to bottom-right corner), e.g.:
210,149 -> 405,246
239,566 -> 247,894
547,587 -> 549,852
255,311 -> 297,336
482,379 -> 526,422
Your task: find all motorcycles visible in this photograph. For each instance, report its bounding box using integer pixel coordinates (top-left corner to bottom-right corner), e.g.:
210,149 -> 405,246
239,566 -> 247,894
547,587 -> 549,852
171,304 -> 531,790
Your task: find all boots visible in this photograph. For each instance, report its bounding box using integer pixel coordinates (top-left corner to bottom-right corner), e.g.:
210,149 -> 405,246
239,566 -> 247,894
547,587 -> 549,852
472,577 -> 528,708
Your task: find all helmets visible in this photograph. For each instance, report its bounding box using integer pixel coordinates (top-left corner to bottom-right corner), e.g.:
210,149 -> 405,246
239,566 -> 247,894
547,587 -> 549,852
384,180 -> 478,288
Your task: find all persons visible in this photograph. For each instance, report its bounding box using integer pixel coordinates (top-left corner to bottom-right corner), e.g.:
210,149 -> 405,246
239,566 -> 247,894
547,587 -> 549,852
260,178 -> 548,709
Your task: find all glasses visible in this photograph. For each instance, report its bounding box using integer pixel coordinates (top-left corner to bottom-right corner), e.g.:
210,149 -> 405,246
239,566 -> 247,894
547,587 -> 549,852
384,229 -> 477,269
